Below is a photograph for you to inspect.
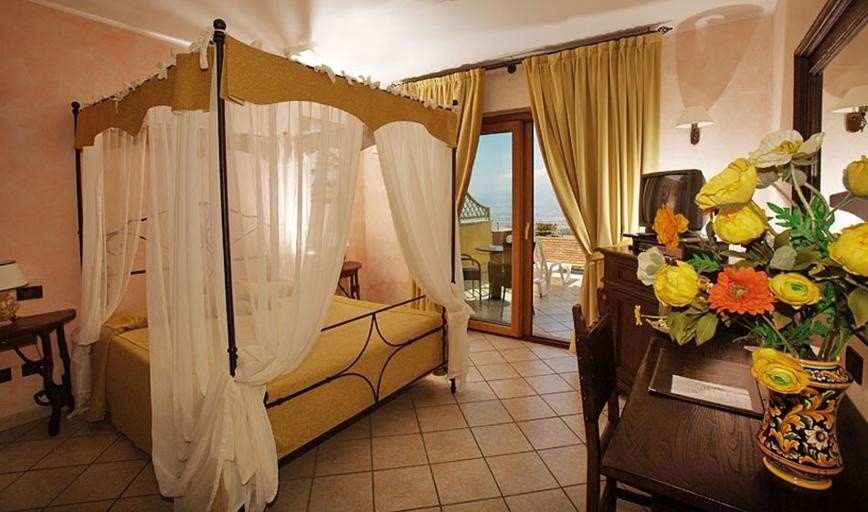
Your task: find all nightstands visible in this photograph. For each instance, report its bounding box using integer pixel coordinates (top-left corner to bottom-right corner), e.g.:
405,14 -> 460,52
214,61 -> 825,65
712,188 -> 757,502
1,307 -> 82,441
338,260 -> 360,302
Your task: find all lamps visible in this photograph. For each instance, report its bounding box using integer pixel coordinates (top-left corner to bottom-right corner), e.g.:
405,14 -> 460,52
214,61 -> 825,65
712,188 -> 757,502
0,259 -> 29,327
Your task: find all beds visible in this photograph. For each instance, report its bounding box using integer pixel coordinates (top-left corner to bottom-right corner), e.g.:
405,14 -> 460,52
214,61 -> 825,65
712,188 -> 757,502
67,17 -> 474,511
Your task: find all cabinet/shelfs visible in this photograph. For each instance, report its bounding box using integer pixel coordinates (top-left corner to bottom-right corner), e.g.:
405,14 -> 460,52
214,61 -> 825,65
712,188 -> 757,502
596,241 -> 661,399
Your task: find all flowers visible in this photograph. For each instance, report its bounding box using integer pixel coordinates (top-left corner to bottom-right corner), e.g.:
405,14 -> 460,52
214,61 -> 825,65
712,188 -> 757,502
635,125 -> 862,396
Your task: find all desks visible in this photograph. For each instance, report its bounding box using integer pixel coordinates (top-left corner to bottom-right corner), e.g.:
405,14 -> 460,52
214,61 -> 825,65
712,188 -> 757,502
600,322 -> 863,512
475,243 -> 514,307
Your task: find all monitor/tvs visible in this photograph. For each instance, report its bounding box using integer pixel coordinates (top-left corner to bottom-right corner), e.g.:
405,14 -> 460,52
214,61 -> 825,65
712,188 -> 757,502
639,169 -> 707,230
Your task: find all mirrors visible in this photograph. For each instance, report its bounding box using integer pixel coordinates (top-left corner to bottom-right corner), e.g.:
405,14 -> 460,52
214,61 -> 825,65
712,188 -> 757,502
791,0 -> 863,238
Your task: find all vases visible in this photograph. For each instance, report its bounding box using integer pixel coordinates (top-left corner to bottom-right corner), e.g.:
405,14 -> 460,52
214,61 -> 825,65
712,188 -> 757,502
751,356 -> 856,495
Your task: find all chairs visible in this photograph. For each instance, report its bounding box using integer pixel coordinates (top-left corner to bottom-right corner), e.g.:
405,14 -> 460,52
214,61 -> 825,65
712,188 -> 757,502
458,253 -> 482,304
533,243 -> 580,297
572,289 -> 653,511
